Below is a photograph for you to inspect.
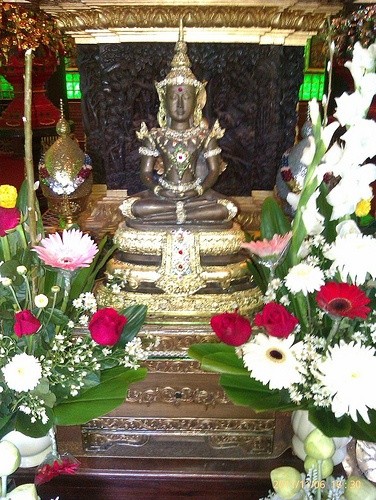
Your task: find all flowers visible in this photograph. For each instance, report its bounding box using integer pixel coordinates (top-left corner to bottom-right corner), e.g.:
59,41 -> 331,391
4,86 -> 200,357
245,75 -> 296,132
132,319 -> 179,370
189,41 -> 375,444
261,428 -> 375,500
0,180 -> 146,486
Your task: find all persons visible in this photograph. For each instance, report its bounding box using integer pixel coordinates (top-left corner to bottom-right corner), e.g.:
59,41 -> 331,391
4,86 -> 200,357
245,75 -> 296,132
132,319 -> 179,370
117,40 -> 240,223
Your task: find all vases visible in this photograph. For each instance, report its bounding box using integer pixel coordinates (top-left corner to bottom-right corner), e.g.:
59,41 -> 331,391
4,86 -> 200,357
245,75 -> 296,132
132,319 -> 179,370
290,405 -> 351,467
2,423 -> 55,470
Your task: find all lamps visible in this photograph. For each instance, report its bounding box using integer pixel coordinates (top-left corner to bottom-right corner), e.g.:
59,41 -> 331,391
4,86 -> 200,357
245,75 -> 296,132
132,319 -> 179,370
0,72 -> 14,112
65,67 -> 81,103
299,69 -> 325,105
